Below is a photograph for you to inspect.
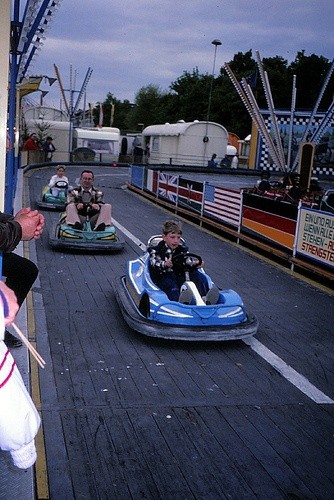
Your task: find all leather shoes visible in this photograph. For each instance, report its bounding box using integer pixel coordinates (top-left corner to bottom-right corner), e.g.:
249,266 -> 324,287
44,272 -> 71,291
4,330 -> 22,347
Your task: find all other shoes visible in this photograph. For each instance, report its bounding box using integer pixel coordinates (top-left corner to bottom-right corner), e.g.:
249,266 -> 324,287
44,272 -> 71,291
178,289 -> 192,305
94,223 -> 105,231
203,287 -> 220,304
74,221 -> 82,231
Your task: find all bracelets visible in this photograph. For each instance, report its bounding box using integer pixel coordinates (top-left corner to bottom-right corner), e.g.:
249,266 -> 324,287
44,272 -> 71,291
1,290 -> 10,319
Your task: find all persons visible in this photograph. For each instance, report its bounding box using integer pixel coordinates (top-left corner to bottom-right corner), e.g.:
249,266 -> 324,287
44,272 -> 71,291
49,165 -> 68,197
208,154 -> 218,168
0,207 -> 44,469
147,221 -> 220,305
66,171 -> 111,231
23,133 -> 56,159
